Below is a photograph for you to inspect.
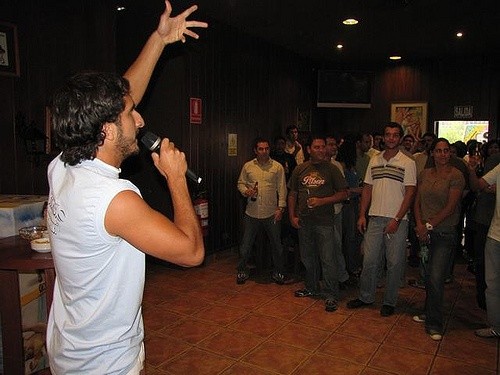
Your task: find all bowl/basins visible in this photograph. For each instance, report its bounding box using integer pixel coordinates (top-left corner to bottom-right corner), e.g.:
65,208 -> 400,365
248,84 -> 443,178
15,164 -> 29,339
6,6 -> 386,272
19,225 -> 47,243
30,237 -> 51,253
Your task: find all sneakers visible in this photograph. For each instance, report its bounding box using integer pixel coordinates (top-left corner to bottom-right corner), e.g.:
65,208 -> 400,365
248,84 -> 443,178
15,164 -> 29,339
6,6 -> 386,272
475,326 -> 500,338
413,314 -> 425,322
428,329 -> 442,340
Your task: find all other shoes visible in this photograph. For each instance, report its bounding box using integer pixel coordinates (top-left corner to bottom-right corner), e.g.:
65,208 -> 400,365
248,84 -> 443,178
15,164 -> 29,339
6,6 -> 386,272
408,257 -> 420,267
347,299 -> 372,308
276,272 -> 286,284
409,279 -> 425,289
338,280 -> 351,289
326,296 -> 337,311
236,273 -> 248,284
381,305 -> 394,316
350,272 -> 360,280
295,288 -> 321,297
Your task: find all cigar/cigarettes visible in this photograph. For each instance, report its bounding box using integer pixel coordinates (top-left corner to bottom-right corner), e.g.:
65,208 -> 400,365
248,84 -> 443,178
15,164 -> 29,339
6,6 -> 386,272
387,233 -> 390,240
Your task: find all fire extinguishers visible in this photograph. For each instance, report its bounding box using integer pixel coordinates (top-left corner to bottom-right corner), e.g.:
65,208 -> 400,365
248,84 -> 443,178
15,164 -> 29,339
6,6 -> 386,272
191,191 -> 209,237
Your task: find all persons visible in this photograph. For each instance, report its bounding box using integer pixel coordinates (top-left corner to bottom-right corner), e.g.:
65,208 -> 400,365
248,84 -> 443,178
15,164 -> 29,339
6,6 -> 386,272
287,135 -> 350,312
238,122 -> 500,340
475,163 -> 500,336
345,122 -> 418,317
45,0 -> 208,375
238,137 -> 287,286
413,138 -> 464,340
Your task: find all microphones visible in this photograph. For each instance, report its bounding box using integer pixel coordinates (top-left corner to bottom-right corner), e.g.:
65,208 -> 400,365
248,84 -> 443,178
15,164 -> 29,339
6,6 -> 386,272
251,181 -> 260,201
140,130 -> 203,183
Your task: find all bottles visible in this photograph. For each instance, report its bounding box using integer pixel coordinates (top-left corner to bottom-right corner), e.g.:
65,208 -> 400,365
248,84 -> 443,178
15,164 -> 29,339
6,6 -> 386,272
251,181 -> 258,201
421,237 -> 432,264
306,189 -> 312,208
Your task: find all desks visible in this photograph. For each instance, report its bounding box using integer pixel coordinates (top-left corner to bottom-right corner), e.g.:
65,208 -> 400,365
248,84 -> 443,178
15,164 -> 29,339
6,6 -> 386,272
0,234 -> 55,375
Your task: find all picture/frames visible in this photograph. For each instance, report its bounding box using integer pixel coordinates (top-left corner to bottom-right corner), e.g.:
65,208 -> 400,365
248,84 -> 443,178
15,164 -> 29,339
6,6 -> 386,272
390,99 -> 428,137
0,21 -> 20,78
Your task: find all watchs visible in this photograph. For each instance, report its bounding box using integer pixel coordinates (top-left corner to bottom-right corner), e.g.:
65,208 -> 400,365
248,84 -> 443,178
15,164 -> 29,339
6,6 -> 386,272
426,222 -> 434,231
395,217 -> 402,224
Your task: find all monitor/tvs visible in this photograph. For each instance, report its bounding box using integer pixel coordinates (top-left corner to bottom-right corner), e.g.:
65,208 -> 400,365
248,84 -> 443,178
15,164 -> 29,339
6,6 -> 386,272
433,116 -> 490,143
